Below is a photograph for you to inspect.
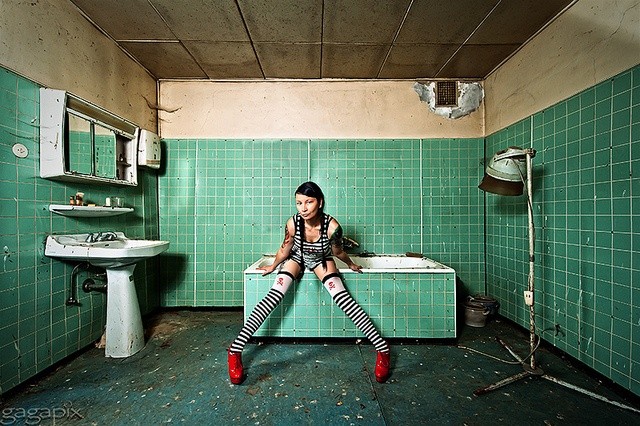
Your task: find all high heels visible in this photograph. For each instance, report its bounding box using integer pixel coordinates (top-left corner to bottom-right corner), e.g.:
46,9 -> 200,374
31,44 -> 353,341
375,346 -> 390,383
228,347 -> 243,384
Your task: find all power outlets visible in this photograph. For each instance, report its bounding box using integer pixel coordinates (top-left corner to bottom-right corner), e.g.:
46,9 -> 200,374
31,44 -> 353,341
12,143 -> 29,158
521,290 -> 535,308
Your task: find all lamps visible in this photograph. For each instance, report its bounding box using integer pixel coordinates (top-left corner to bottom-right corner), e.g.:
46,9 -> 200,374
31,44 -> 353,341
456,144 -> 640,415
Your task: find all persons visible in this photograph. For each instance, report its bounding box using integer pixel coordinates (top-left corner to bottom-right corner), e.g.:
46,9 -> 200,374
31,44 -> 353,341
226,181 -> 392,384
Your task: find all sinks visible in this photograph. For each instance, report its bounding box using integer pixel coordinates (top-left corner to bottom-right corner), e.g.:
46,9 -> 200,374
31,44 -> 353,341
84,240 -> 163,248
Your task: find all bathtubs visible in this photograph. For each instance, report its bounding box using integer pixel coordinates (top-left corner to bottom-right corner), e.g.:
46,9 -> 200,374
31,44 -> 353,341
245,254 -> 456,339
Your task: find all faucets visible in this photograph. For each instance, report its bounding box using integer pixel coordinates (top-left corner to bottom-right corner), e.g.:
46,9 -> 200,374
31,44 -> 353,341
106,231 -> 117,239
